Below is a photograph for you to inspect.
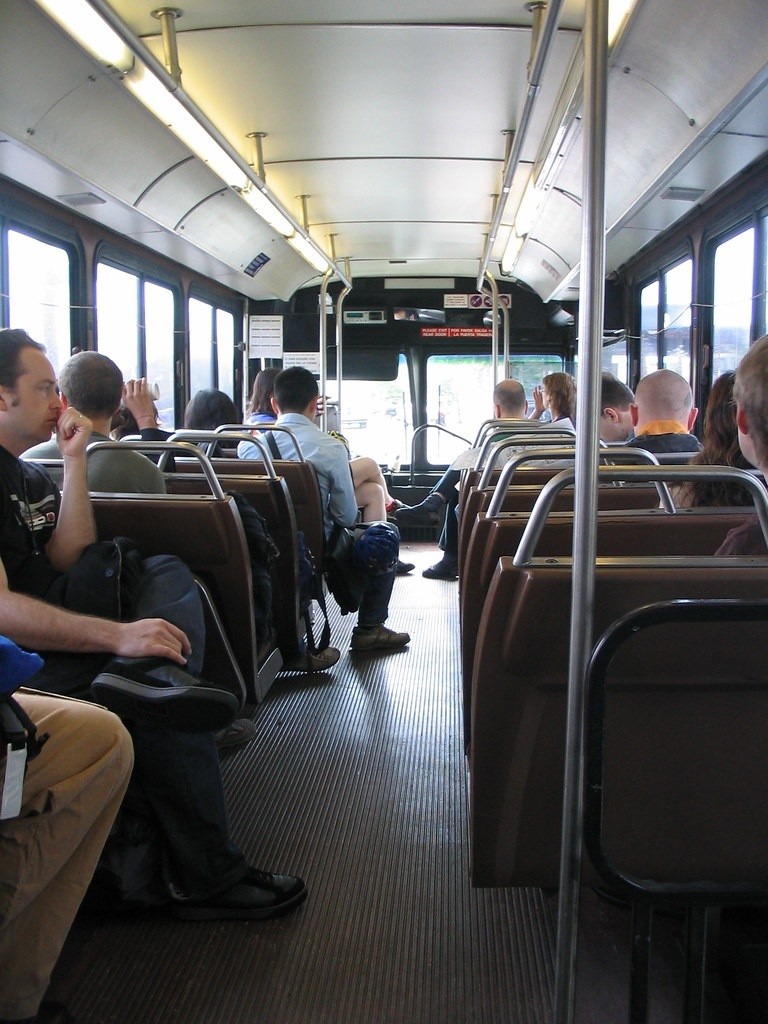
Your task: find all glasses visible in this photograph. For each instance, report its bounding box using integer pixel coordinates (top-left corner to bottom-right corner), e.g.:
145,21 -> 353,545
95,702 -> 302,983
540,390 -> 546,394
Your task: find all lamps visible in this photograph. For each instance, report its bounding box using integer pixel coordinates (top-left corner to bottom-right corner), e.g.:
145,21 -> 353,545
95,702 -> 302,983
29,0 -> 330,275
499,0 -> 644,277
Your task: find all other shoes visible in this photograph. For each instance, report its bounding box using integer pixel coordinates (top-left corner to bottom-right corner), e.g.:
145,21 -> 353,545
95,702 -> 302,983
423,567 -> 456,582
393,503 -> 440,528
388,498 -> 413,517
396,560 -> 415,574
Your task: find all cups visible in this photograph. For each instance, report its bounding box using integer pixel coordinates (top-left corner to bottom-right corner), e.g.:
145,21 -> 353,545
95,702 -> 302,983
121,383 -> 160,408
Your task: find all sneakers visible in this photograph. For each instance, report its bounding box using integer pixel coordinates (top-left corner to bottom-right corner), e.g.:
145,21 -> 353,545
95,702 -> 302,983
213,718 -> 256,750
277,645 -> 340,680
350,625 -> 410,651
89,655 -> 240,731
164,839 -> 308,923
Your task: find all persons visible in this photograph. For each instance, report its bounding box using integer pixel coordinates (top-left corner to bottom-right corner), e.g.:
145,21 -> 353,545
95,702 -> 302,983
17,350 -> 704,751
712,334 -> 768,556
0,328 -> 308,920
0,559 -> 192,1024
661,369 -> 759,509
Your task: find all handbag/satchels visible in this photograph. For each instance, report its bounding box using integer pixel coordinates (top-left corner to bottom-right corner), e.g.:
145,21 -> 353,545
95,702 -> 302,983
322,525 -> 364,616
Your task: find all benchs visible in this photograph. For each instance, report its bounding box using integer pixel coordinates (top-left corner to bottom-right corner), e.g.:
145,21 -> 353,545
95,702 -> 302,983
461,418 -> 768,1023
22,423 -> 326,719
